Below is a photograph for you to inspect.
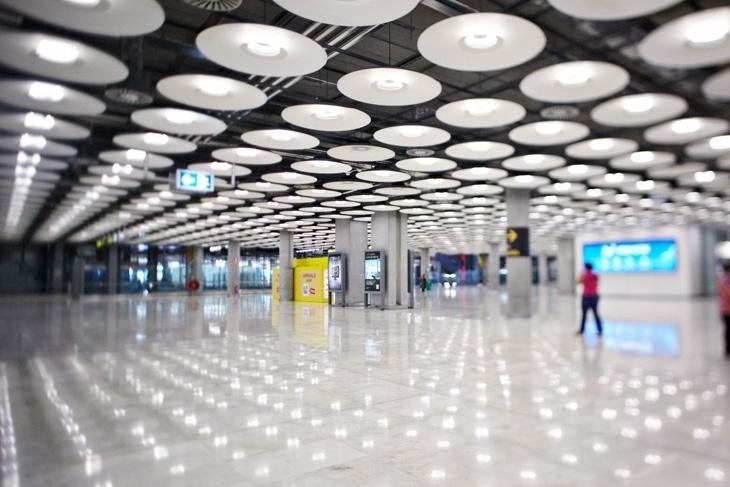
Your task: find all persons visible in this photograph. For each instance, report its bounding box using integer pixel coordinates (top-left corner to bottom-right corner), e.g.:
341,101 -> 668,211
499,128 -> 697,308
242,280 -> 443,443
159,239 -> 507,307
576,263 -> 601,334
716,262 -> 730,358
420,274 -> 427,294
